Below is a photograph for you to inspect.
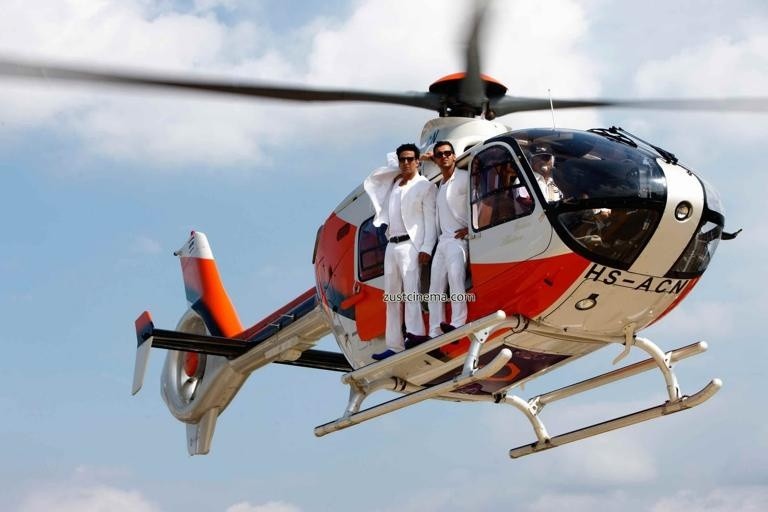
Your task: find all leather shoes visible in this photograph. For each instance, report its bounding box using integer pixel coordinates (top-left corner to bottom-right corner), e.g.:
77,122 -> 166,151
371,349 -> 397,361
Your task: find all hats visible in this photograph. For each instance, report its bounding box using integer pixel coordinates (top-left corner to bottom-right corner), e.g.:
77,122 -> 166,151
528,142 -> 554,159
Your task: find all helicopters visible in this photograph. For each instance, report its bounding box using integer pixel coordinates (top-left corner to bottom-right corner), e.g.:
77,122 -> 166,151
0,0 -> 768,458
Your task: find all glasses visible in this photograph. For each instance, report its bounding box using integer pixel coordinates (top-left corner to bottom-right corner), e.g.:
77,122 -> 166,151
434,150 -> 455,158
398,156 -> 417,162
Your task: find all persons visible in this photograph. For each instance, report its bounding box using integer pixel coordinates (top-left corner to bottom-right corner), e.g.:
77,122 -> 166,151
363,141 -> 438,361
574,193 -> 612,222
512,144 -> 603,246
406,141 -> 469,340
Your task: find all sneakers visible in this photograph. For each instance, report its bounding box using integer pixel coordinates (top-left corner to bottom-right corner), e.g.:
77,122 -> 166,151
407,332 -> 439,356
439,321 -> 460,345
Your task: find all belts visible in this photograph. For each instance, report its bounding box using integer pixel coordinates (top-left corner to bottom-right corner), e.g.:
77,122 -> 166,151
389,234 -> 411,243
